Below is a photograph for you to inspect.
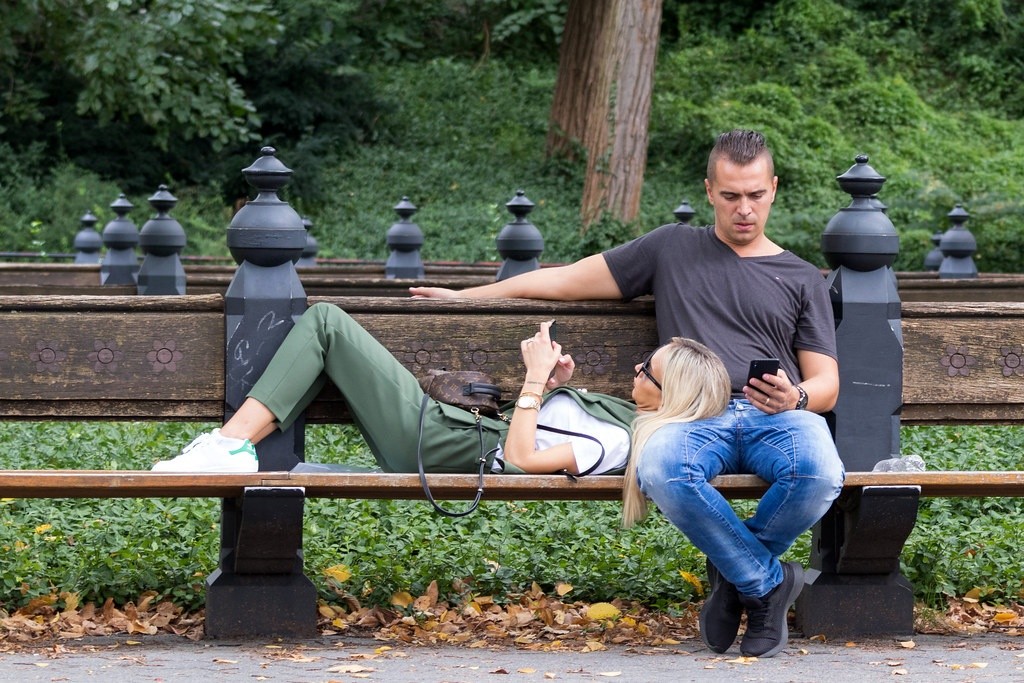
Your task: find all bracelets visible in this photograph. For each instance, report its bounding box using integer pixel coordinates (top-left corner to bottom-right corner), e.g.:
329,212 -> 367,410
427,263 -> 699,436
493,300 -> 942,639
524,381 -> 546,385
519,391 -> 543,404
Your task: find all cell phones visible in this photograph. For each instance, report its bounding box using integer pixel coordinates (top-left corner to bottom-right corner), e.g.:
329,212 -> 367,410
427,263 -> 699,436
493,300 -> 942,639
549,319 -> 556,346
747,359 -> 780,396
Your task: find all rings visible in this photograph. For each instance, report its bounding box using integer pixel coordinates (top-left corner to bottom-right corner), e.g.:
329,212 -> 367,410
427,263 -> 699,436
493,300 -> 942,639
765,398 -> 769,405
527,340 -> 534,344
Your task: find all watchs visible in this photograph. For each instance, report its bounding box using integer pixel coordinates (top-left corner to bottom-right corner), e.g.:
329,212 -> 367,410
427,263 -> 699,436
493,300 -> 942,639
795,386 -> 808,410
515,395 -> 541,412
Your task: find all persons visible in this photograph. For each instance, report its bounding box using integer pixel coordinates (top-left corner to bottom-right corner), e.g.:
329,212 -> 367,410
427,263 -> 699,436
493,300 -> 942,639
153,301 -> 732,530
409,129 -> 846,658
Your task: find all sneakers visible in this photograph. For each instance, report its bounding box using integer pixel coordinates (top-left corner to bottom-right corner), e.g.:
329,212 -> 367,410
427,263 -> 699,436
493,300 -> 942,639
740,560 -> 805,658
150,428 -> 260,474
699,557 -> 744,654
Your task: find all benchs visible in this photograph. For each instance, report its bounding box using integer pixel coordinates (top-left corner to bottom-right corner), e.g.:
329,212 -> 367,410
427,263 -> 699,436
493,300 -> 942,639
0,147 -> 1024,647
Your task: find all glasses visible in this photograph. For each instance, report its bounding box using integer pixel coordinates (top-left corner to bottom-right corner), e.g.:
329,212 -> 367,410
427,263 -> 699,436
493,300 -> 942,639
636,344 -> 665,391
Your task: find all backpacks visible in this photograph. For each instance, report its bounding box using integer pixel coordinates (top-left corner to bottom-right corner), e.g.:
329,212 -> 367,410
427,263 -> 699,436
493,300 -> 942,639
418,369 -> 604,517
